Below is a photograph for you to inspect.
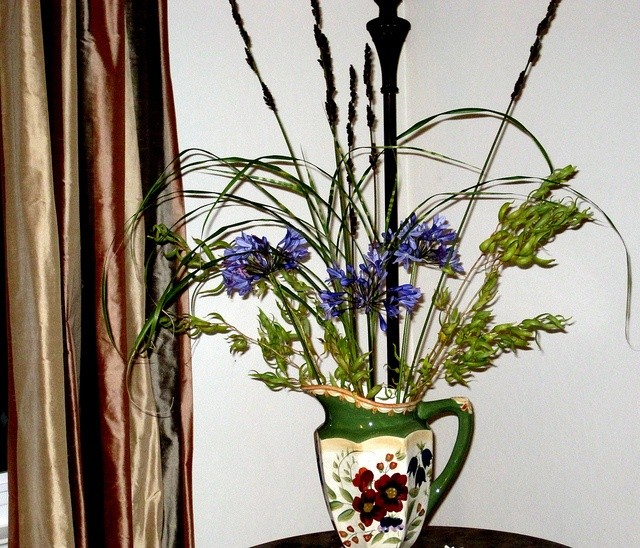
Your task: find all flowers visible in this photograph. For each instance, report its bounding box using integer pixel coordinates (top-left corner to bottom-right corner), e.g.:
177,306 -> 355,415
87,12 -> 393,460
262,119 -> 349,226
97,2 -> 633,415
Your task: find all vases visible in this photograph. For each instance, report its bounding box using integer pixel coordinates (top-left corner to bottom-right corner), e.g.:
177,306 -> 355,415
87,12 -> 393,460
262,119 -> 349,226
302,386 -> 474,548
